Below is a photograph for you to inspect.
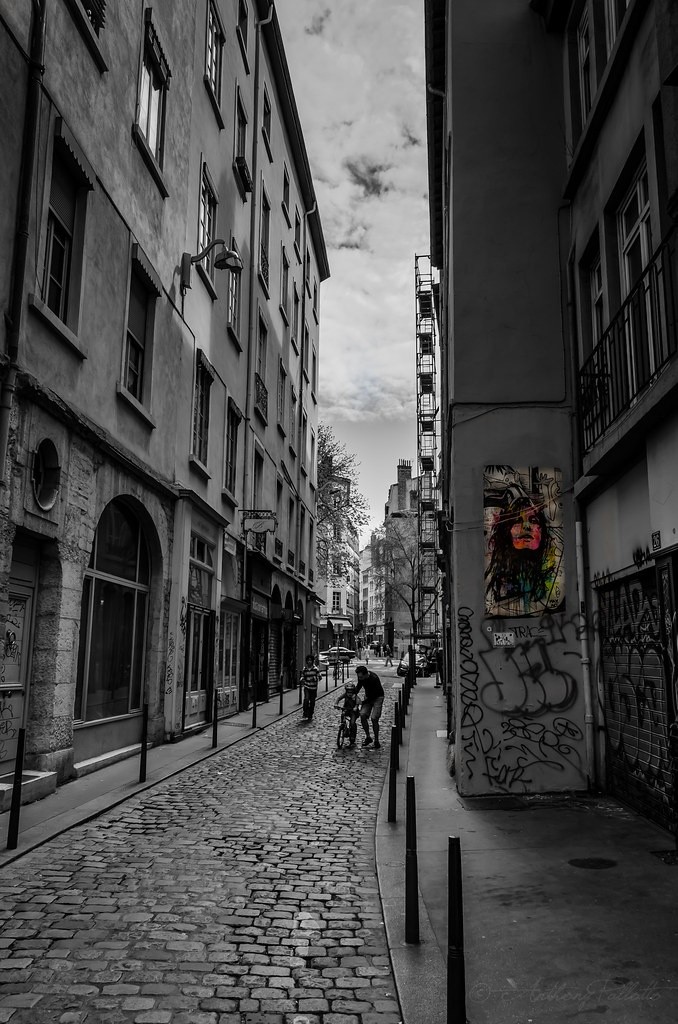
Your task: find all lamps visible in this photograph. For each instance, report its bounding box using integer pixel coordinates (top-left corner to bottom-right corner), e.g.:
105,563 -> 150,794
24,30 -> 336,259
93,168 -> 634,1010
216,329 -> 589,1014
181,239 -> 244,298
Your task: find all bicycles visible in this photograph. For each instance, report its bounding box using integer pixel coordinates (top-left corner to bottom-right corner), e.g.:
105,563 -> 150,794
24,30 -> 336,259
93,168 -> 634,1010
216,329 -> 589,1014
334,704 -> 358,749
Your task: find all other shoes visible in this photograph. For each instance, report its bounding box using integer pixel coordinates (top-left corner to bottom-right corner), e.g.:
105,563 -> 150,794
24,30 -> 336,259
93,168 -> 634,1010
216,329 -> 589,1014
362,736 -> 373,745
302,715 -> 308,718
308,716 -> 312,722
374,741 -> 381,748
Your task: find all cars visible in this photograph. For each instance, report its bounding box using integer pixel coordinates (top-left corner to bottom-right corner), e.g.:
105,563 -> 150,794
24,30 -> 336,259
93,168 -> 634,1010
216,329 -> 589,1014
397,651 -> 423,677
319,653 -> 330,672
370,641 -> 379,650
431,646 -> 443,661
320,647 -> 356,664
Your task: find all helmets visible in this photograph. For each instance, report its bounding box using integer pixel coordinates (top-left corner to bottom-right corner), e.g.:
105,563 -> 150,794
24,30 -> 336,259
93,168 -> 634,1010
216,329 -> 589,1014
344,682 -> 356,691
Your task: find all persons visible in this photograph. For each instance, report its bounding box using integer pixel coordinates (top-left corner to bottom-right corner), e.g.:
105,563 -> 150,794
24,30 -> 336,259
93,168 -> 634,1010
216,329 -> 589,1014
375,642 -> 381,657
362,646 -> 370,665
383,643 -> 394,667
353,666 -> 385,748
299,654 -> 323,722
334,683 -> 363,750
